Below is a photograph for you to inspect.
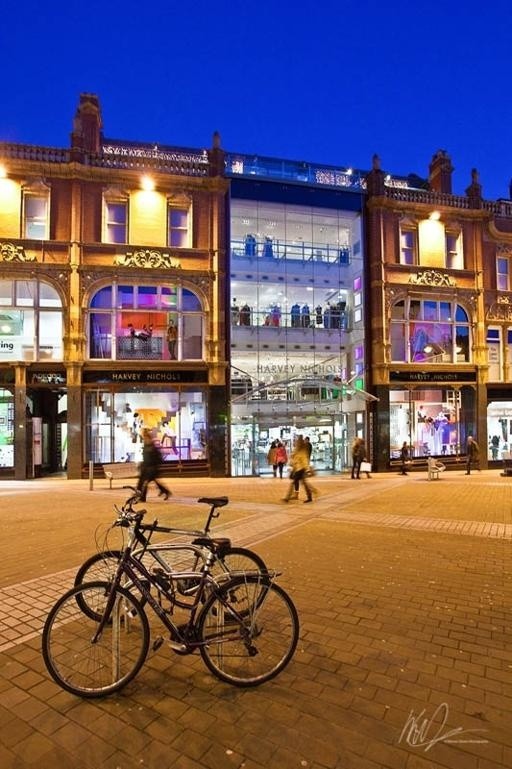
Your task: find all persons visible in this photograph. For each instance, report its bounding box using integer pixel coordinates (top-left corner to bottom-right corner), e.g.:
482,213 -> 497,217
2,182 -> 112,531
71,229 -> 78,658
128,427 -> 166,498
264,434 -> 372,505
232,232 -> 349,330
161,421 -> 179,454
400,441 -> 409,475
393,404 -> 456,454
166,319 -> 177,360
133,436 -> 173,504
464,435 -> 481,475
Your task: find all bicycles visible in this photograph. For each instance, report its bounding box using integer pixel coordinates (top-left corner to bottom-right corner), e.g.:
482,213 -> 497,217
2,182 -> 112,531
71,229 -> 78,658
73,484 -> 274,626
42,509 -> 300,697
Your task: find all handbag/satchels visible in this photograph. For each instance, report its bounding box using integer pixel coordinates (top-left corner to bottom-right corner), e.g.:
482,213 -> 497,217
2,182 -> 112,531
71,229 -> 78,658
360,461 -> 372,472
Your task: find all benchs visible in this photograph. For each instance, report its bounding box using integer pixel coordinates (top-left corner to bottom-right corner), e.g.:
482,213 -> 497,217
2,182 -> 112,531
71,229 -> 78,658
103,463 -> 140,490
426,457 -> 446,481
